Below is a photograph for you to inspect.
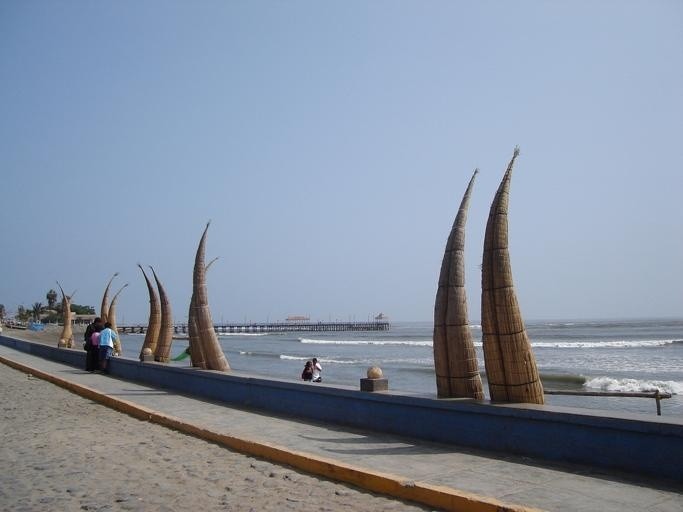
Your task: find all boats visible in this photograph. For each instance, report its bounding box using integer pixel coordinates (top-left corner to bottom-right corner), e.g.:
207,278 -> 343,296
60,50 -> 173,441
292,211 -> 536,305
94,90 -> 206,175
29,321 -> 43,330
6,321 -> 27,328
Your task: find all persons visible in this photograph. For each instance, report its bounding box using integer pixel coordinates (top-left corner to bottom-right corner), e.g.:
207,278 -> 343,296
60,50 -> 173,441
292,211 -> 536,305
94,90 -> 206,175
312,358 -> 322,382
302,361 -> 313,381
89,327 -> 100,374
97,321 -> 117,374
84,317 -> 101,370
